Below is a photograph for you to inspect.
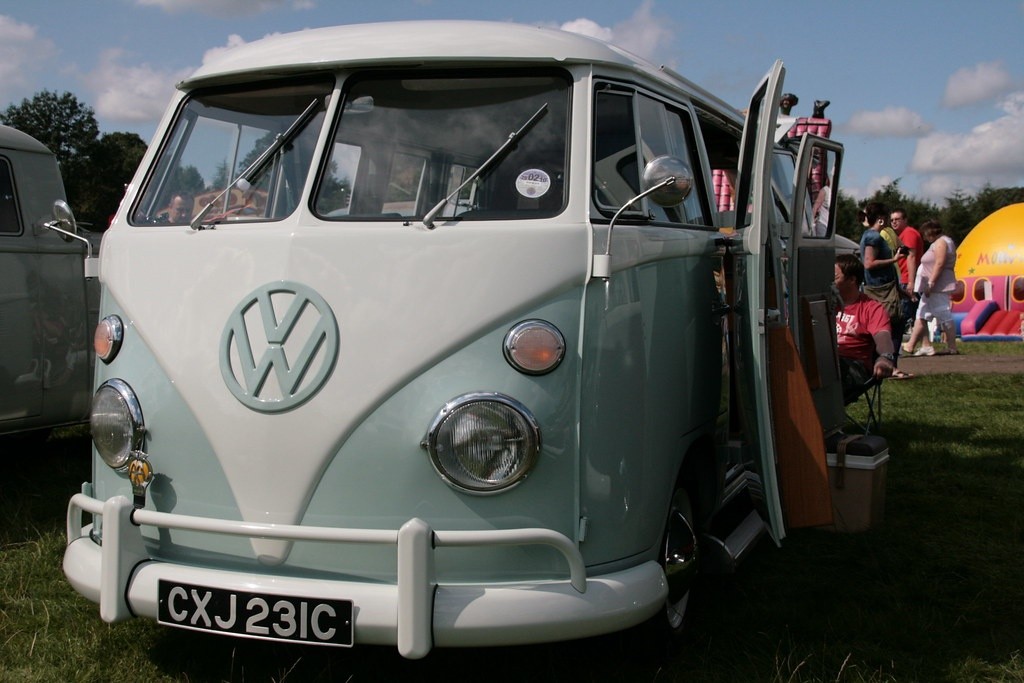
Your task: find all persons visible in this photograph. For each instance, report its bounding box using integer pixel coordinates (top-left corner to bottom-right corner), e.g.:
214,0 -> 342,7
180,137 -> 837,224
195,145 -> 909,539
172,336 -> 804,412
809,185 -> 834,238
830,254 -> 894,426
860,201 -> 960,380
154,192 -> 195,224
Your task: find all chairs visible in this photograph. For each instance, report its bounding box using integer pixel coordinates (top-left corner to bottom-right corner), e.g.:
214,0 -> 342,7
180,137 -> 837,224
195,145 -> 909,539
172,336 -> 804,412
845,317 -> 905,437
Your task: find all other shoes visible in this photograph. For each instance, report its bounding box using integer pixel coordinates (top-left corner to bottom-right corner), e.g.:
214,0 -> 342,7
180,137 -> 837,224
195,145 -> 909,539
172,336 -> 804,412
935,350 -> 959,355
913,345 -> 935,356
901,342 -> 914,354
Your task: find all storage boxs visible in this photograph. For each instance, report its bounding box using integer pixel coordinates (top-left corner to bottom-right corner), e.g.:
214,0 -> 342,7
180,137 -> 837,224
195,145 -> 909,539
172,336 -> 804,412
812,437 -> 889,534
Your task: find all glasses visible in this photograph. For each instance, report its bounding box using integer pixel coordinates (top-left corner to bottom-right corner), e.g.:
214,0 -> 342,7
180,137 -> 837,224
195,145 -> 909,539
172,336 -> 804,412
890,218 -> 904,222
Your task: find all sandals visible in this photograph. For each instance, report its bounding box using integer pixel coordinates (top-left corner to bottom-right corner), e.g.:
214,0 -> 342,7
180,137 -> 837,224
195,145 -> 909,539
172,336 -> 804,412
884,366 -> 915,380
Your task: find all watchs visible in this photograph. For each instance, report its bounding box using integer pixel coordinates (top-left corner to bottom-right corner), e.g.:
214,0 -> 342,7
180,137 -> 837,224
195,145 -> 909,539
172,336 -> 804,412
880,353 -> 894,361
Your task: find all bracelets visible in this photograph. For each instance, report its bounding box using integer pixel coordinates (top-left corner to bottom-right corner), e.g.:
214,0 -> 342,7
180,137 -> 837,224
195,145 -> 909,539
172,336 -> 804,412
927,282 -> 935,285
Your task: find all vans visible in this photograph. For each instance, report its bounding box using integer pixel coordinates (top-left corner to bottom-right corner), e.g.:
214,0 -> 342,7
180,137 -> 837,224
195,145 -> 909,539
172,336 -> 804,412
42,15 -> 847,659
0,121 -> 98,439
